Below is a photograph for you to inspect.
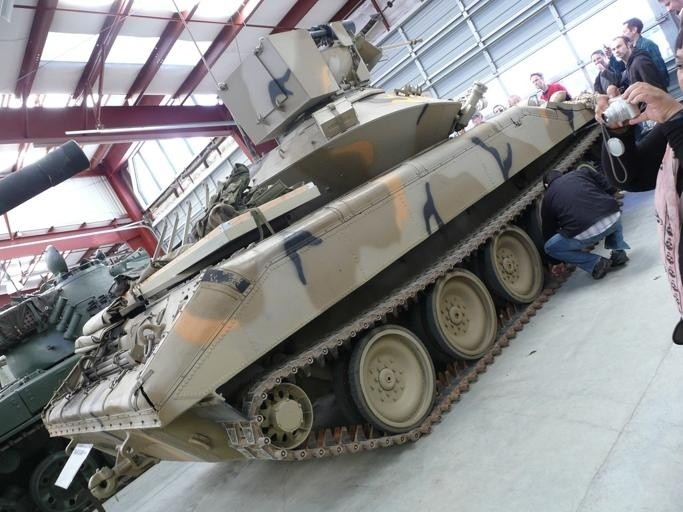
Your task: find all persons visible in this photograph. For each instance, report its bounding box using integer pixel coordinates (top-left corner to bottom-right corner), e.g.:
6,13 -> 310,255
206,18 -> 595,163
591,18 -> 669,140
493,105 -> 506,116
541,167 -> 629,279
531,73 -> 572,100
595,30 -> 682,345
509,95 -> 521,106
472,113 -> 484,127
659,0 -> 683,14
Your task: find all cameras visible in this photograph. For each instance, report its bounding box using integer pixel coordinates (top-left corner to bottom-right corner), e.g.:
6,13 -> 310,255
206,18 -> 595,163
600,93 -> 640,124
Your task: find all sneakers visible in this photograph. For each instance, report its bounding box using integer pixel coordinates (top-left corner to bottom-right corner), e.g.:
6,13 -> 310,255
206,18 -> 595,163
592,257 -> 612,279
610,250 -> 629,267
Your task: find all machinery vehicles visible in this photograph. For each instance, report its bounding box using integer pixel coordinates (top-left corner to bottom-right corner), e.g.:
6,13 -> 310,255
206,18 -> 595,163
43,19 -> 625,464
2,141 -> 168,511
